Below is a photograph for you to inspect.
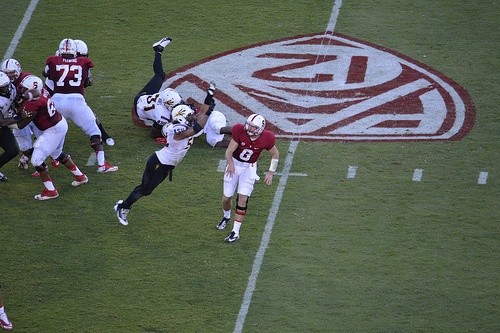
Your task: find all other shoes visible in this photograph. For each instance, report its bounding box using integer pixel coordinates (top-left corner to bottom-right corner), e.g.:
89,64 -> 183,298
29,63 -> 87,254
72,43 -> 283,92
0,318 -> 13,331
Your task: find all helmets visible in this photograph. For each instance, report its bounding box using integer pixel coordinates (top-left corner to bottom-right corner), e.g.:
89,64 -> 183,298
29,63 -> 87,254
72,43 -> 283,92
245,114 -> 266,135
162,88 -> 181,110
74,40 -> 89,55
2,59 -> 22,82
0,72 -> 10,95
20,75 -> 43,99
58,38 -> 77,58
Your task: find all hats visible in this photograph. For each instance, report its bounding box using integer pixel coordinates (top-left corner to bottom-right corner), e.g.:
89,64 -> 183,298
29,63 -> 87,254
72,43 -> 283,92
171,104 -> 195,129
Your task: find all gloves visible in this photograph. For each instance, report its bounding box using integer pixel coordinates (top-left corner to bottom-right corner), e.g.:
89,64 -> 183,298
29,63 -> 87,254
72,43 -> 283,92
207,79 -> 215,96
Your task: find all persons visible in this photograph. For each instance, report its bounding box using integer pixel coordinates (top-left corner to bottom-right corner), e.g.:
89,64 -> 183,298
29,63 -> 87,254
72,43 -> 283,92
0,59 -> 88,200
18,38 -> 118,172
216,114 -> 279,241
114,82 -> 216,226
134,38 -> 232,147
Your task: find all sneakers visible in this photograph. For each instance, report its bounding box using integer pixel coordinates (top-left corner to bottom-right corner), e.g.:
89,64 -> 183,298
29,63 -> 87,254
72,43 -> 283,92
216,217 -> 230,230
71,173 -> 89,186
96,161 -> 118,172
35,187 -> 59,201
152,137 -> 166,144
223,231 -> 240,243
152,37 -> 172,50
18,159 -> 28,168
32,165 -> 48,177
113,200 -> 130,225
50,158 -> 59,168
0,172 -> 8,182
105,138 -> 115,146
